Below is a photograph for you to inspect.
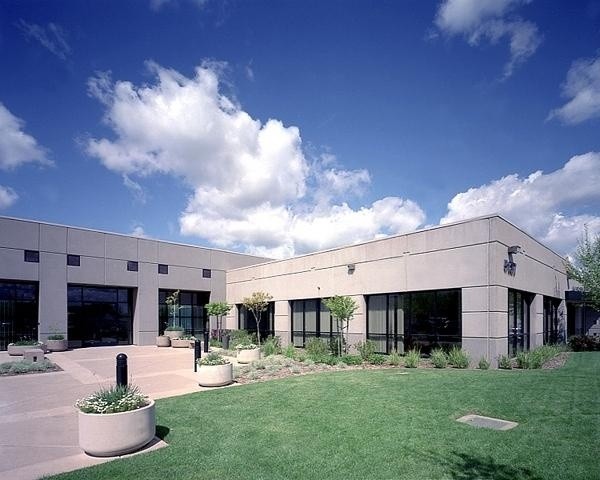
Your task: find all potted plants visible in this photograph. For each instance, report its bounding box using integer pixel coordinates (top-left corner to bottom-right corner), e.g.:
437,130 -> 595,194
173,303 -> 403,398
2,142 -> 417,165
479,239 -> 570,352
8,337 -> 44,356
196,352 -> 233,387
164,325 -> 183,338
75,372 -> 157,456
46,327 -> 68,351
236,334 -> 261,364
